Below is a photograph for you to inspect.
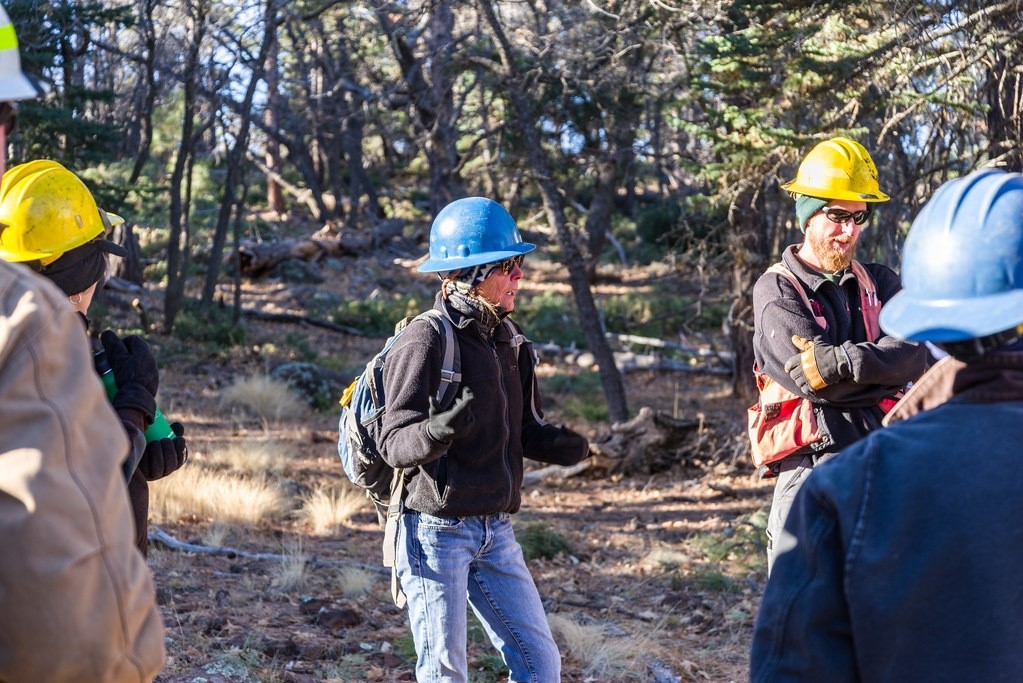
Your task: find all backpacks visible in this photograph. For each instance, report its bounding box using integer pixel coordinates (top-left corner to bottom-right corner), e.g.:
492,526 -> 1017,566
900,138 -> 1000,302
338,310 -> 520,499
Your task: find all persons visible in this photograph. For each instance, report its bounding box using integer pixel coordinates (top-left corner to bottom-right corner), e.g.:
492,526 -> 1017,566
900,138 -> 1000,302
380,197 -> 589,683
0,160 -> 190,562
751,165 -> 1023,683
750,135 -> 927,577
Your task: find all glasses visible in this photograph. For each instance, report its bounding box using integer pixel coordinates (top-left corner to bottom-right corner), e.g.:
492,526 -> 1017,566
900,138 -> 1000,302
494,255 -> 524,269
2,106 -> 15,133
820,206 -> 870,225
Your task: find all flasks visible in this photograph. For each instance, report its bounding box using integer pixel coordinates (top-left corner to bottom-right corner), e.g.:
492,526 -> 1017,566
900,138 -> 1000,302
93,349 -> 178,450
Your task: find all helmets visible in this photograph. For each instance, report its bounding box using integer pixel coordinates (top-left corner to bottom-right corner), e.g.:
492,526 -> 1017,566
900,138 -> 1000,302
1,160 -> 125,262
880,168 -> 1023,340
1,5 -> 37,99
415,196 -> 536,271
781,138 -> 892,202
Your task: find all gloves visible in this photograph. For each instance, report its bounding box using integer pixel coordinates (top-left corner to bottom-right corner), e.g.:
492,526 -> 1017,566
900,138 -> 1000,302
425,387 -> 475,441
550,426 -> 588,467
102,329 -> 156,423
785,336 -> 852,394
141,422 -> 185,481
